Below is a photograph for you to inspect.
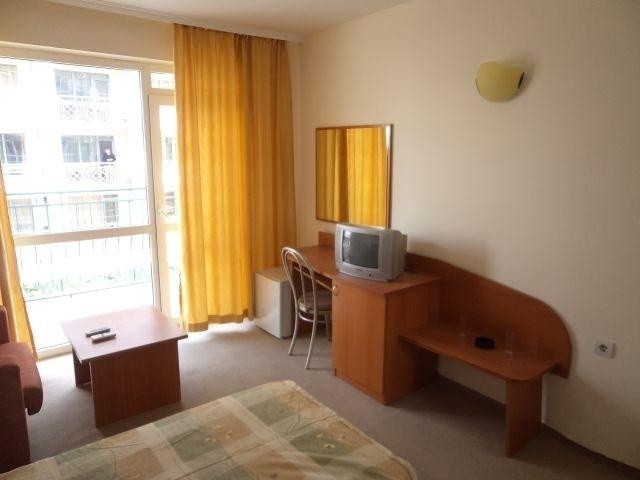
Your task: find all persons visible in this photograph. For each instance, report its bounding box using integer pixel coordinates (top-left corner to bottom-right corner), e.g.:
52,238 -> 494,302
103,149 -> 116,162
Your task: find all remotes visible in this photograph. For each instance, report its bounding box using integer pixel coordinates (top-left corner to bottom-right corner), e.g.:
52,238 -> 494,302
90,331 -> 117,342
84,327 -> 110,337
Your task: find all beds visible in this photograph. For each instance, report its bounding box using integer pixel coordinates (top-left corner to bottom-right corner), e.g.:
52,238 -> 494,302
0,376 -> 416,479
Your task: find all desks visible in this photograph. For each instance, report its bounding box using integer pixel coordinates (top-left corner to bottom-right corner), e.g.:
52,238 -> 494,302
62,304 -> 188,431
288,245 -> 575,460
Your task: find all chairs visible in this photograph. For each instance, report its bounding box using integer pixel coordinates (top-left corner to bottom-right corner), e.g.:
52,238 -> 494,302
280,246 -> 331,369
0,305 -> 44,477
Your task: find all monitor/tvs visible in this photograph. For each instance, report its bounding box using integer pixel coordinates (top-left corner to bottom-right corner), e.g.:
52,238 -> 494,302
334,222 -> 408,282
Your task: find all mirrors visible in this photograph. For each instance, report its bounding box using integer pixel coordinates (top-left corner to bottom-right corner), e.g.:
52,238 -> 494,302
315,123 -> 394,230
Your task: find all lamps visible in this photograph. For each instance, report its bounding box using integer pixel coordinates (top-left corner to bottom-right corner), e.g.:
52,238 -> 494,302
476,54 -> 531,104
